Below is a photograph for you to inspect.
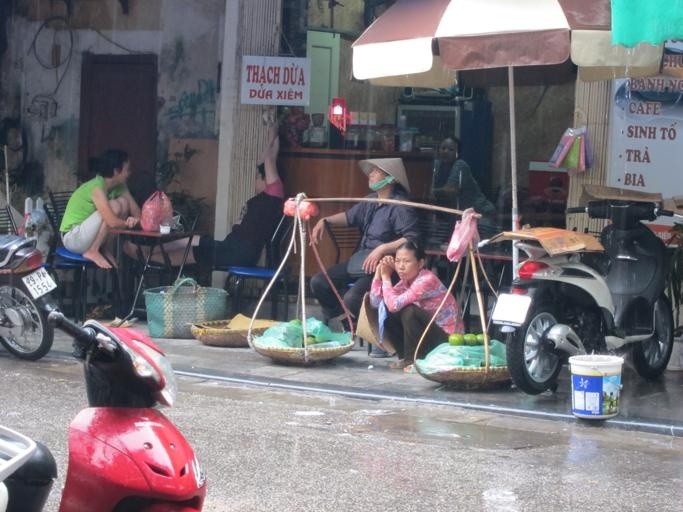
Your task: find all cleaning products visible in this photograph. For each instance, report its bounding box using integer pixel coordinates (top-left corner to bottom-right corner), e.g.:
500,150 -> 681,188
398,110 -> 406,128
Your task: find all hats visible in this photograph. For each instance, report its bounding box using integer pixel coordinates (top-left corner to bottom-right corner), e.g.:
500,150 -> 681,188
357,157 -> 410,191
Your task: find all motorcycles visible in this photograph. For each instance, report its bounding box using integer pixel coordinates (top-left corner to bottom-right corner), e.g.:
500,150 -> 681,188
1,228 -> 60,361
489,198 -> 675,399
0,303 -> 206,512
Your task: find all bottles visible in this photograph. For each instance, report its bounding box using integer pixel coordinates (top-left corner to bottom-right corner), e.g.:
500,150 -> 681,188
304,341 -> 340,351
347,122 -> 420,153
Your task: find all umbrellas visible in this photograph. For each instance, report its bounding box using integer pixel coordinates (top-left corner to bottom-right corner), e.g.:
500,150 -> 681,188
352,0 -> 683,286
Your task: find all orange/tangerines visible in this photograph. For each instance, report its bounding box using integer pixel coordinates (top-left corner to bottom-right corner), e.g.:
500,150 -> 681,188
449,333 -> 490,346
303,335 -> 326,345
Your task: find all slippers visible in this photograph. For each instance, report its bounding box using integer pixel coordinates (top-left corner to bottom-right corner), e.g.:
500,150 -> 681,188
104,316 -> 135,329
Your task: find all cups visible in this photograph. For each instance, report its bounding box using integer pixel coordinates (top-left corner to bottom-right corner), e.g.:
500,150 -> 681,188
311,113 -> 325,128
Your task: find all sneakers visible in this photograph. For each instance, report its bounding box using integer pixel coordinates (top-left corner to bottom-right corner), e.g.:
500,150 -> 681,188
369,346 -> 388,358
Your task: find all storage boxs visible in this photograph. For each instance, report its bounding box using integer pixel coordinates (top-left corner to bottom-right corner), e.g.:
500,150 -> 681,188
528,159 -> 569,203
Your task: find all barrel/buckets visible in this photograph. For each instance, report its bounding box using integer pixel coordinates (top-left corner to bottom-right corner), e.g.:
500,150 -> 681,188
568,354 -> 625,421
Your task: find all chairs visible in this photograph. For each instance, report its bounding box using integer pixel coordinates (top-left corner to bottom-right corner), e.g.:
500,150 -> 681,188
37,181 -> 302,325
0,205 -> 40,305
320,214 -> 532,337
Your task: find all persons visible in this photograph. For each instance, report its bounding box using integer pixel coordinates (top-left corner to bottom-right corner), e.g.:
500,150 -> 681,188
58,149 -> 141,270
123,128 -> 287,267
369,239 -> 465,373
308,157 -> 426,338
428,136 -> 496,237
